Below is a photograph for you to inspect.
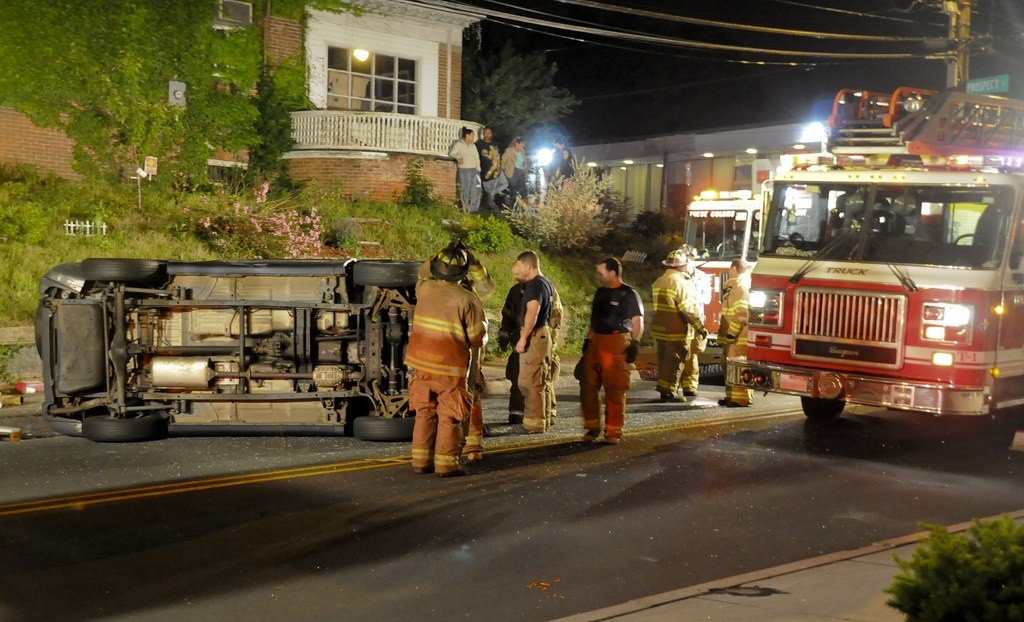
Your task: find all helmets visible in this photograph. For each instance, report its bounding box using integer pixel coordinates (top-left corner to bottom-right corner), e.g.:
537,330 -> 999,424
430,247 -> 469,282
677,244 -> 700,260
662,250 -> 688,267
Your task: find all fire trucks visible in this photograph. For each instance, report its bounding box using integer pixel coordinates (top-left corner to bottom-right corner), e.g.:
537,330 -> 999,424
634,190 -> 988,382
725,86 -> 1024,420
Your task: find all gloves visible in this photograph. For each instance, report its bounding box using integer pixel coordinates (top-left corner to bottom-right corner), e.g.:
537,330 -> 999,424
446,238 -> 476,265
497,335 -> 509,352
624,340 -> 639,364
582,338 -> 592,355
726,334 -> 735,339
701,332 -> 707,339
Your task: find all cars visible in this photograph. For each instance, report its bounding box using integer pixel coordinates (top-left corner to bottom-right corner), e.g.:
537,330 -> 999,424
33,258 -> 424,441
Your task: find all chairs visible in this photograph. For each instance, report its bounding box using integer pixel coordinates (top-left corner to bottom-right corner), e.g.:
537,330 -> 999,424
865,233 -> 994,268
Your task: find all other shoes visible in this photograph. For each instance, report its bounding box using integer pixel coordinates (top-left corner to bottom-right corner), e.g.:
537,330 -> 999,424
718,398 -> 730,406
606,436 -> 620,444
511,425 -> 537,434
661,391 -> 687,402
434,465 -> 469,476
727,401 -> 741,407
584,429 -> 600,441
467,451 -> 482,460
683,392 -> 697,396
509,411 -> 523,424
550,415 -> 556,424
413,461 -> 433,473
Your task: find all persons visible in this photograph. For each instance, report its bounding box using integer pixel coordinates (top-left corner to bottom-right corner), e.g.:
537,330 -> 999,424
403,237 -> 489,478
580,256 -> 645,445
649,243 -> 712,403
716,259 -> 755,408
448,126 -> 576,215
495,250 -> 563,434
